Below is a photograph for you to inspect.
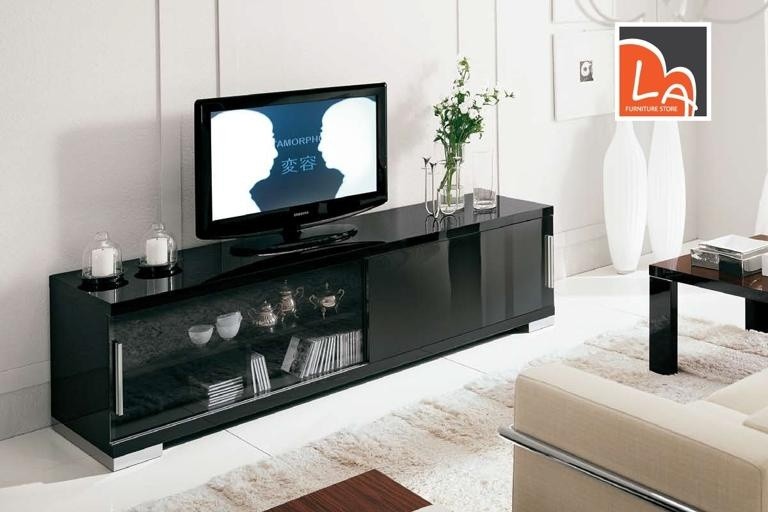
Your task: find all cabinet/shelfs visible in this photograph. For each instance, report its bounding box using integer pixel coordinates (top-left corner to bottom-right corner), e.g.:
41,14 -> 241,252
49,192 -> 554,471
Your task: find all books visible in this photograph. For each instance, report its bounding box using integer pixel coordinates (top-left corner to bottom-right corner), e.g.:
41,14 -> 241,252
186,328 -> 365,412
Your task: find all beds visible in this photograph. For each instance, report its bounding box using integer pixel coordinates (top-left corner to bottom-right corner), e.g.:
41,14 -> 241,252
649,234 -> 768,375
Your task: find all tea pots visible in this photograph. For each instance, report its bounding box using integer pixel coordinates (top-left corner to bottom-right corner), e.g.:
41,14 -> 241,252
248,278 -> 345,330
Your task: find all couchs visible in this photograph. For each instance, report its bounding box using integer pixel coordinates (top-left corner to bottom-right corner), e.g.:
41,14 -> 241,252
498,366 -> 768,512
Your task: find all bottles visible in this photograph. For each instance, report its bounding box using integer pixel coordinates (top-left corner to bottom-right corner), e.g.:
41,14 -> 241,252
84,231 -> 124,286
139,224 -> 178,273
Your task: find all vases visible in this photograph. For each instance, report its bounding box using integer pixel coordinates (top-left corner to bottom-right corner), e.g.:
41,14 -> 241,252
436,160 -> 461,216
603,109 -> 688,273
471,150 -> 498,210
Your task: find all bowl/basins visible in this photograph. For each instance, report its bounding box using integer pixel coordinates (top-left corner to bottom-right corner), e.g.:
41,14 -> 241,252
189,325 -> 213,346
215,310 -> 243,341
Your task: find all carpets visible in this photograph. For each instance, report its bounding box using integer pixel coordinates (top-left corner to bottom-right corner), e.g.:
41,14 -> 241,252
116,309 -> 768,512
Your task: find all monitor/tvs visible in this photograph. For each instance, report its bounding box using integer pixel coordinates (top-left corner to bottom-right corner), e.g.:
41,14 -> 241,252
194,83 -> 387,257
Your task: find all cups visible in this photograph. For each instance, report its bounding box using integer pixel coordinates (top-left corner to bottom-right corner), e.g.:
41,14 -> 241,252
470,143 -> 499,213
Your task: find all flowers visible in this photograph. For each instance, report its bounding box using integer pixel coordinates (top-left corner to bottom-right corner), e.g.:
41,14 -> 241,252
430,55 -> 520,215
